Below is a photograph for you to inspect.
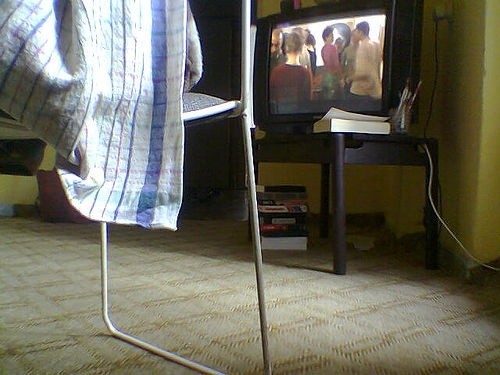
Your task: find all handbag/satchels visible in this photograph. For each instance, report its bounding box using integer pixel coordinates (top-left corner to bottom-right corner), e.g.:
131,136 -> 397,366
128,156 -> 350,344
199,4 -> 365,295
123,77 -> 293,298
37,170 -> 97,223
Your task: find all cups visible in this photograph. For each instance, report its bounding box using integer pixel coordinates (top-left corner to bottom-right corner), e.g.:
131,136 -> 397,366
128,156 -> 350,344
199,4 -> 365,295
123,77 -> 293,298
392,112 -> 412,136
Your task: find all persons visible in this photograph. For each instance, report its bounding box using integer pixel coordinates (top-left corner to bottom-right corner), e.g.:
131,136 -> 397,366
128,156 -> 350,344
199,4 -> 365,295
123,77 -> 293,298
335,37 -> 344,54
291,27 -> 311,71
346,21 -> 382,100
271,28 -> 283,59
269,31 -> 311,103
341,29 -> 360,74
321,28 -> 345,100
305,34 -> 316,74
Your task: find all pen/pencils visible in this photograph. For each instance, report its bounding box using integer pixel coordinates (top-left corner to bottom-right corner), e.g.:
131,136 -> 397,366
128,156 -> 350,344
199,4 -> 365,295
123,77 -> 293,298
391,80 -> 421,131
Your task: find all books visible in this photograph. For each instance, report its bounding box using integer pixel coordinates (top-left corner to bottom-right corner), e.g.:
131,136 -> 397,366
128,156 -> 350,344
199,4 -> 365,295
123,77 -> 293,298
312,106 -> 392,135
256,185 -> 309,250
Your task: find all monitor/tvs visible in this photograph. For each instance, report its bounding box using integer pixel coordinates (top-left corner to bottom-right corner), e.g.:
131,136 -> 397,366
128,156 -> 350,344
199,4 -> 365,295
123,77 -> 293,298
253,0 -> 424,143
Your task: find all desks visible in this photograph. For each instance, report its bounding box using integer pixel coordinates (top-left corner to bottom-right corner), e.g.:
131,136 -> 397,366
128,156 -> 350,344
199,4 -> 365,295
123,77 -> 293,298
246,134 -> 440,275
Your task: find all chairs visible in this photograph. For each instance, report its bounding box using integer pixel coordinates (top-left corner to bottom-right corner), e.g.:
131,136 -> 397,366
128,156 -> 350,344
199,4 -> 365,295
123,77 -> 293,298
100,0 -> 273,375
